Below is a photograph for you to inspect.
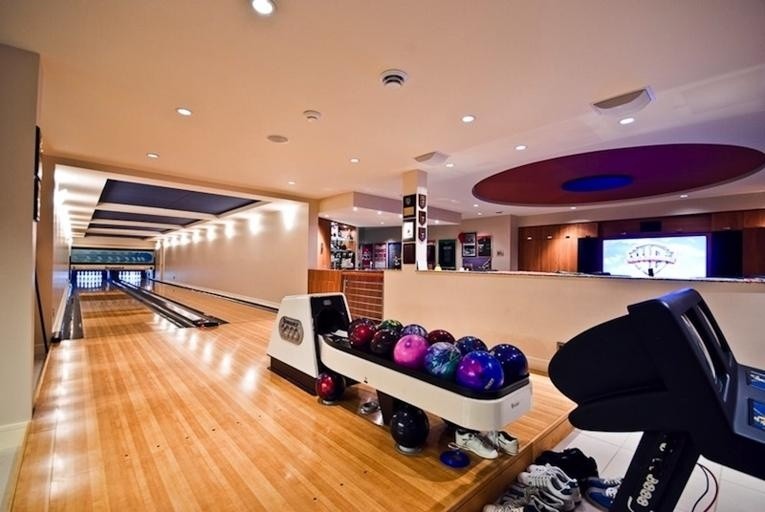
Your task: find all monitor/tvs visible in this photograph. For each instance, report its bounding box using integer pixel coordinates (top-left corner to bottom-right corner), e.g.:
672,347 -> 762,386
596,231 -> 712,279
681,302 -> 723,394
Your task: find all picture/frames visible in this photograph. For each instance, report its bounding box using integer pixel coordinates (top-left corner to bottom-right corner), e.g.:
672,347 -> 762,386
462,232 -> 477,257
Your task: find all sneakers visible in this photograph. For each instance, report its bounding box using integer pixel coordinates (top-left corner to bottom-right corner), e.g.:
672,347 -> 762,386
585,477 -> 624,510
483,448 -> 598,512
478,430 -> 520,457
455,430 -> 498,459
